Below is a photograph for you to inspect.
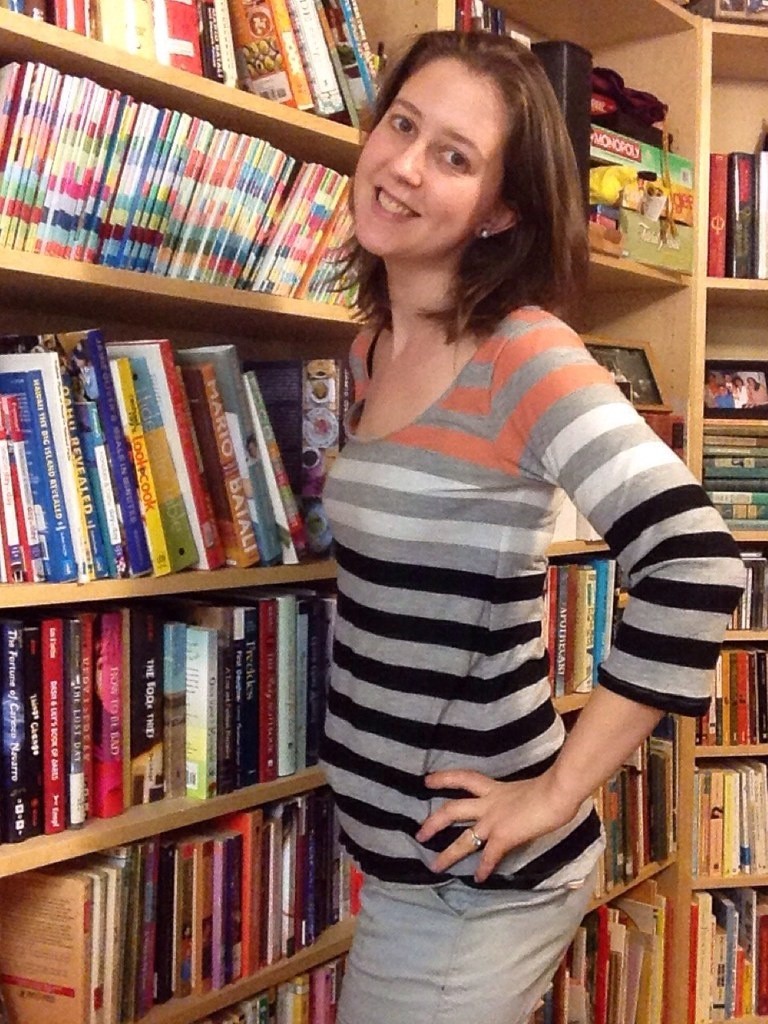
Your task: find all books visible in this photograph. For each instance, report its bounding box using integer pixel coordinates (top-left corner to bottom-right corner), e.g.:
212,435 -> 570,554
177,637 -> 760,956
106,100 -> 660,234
1,0 -> 768,1023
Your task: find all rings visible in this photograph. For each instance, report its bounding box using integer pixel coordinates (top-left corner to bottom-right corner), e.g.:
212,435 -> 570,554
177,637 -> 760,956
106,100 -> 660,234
465,827 -> 484,849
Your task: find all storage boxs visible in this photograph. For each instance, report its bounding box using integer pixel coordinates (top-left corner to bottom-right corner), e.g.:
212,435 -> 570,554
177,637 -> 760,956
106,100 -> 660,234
589,203 -> 620,229
639,178 -> 694,226
617,207 -> 694,276
553,496 -> 604,541
590,122 -> 694,189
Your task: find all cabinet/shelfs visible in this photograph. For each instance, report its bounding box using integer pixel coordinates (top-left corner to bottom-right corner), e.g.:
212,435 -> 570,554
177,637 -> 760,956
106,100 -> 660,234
0,0 -> 768,1024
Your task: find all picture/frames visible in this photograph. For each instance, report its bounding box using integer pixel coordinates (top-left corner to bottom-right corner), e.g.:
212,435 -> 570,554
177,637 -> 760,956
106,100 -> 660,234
579,333 -> 674,413
704,359 -> 768,420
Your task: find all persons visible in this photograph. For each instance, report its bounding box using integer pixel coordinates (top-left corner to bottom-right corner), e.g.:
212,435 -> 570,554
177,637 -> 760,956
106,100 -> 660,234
706,359 -> 768,412
319,29 -> 744,1017
